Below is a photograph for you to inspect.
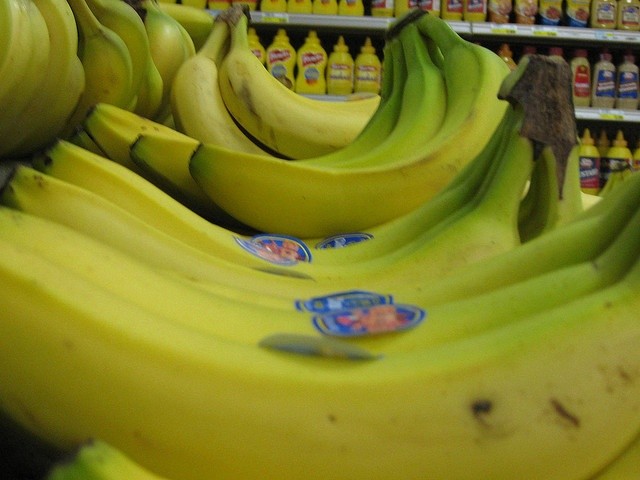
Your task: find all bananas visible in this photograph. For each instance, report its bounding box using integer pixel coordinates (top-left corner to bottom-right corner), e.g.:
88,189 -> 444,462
61,0 -> 150,141
0,53 -> 583,318
0,0 -> 85,158
46,436 -> 163,479
81,9 -> 511,241
597,164 -> 634,197
0,165 -> 640,479
123,0 -> 213,121
171,5 -> 381,162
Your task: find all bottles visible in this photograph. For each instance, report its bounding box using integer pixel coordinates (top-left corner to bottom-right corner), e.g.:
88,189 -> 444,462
632,148 -> 640,174
327,37 -> 355,97
488,0 -> 514,23
591,0 -> 618,31
618,54 -> 640,111
442,0 -> 464,21
570,48 -> 591,106
261,0 -> 287,13
564,0 -> 591,27
394,0 -> 419,19
465,0 -> 488,21
246,27 -> 265,63
313,0 -> 338,15
607,132 -> 632,187
372,0 -> 394,18
337,0 -> 364,15
592,53 -> 617,108
514,0 -> 539,26
617,0 -> 640,32
497,43 -> 517,70
549,47 -> 566,62
233,0 -> 256,12
295,31 -> 327,98
355,38 -> 382,94
519,46 -> 538,64
182,0 -> 204,8
208,0 -> 228,11
539,0 -> 564,26
288,1 -> 313,13
265,29 -> 295,91
596,131 -> 610,190
578,129 -> 600,196
419,1 -> 442,18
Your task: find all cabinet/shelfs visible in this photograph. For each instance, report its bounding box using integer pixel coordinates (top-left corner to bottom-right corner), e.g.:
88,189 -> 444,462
203,10 -> 640,122
